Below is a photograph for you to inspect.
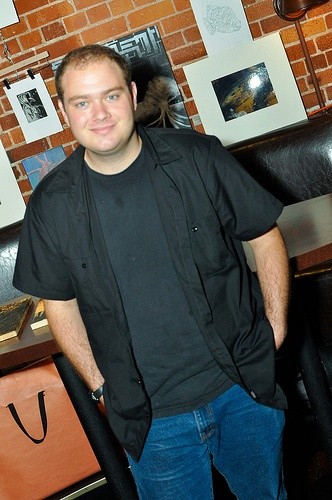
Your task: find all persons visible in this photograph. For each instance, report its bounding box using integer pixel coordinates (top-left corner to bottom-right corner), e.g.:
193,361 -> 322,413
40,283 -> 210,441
13,44 -> 290,500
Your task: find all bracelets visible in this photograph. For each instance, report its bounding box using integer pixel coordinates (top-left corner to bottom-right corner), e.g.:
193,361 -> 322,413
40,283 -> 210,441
91,383 -> 104,402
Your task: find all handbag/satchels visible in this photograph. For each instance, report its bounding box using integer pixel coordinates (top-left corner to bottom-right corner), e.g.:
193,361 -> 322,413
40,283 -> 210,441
1,357 -> 102,500
